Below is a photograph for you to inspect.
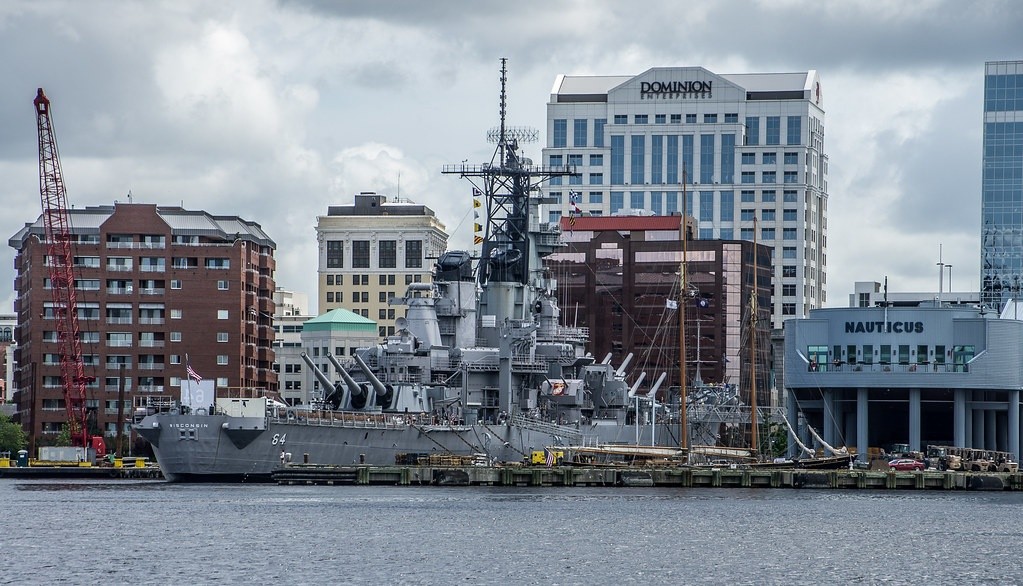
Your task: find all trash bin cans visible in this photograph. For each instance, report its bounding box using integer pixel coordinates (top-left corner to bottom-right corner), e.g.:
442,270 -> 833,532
16,449 -> 28,467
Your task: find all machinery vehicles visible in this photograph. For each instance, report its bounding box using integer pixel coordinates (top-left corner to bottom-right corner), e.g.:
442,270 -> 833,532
32,85 -> 107,459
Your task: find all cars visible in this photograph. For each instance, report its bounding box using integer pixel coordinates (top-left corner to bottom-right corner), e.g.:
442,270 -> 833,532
888,459 -> 926,472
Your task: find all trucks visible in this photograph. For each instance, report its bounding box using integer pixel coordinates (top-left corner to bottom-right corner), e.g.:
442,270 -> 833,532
926,445 -> 1019,474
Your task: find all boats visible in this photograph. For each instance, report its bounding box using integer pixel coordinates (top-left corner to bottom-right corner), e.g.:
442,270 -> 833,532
132,54 -> 791,485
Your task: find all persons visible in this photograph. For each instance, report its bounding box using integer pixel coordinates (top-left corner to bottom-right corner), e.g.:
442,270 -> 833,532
280,449 -> 284,463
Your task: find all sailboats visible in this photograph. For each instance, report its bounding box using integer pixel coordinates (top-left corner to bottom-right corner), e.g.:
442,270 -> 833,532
692,216 -> 859,470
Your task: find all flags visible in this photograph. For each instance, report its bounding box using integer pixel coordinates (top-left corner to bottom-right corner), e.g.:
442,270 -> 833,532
544,448 -> 554,468
570,189 -> 582,226
473,187 -> 483,246
186,363 -> 202,385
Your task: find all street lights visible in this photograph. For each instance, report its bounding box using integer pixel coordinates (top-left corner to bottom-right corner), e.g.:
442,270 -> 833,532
944,264 -> 953,293
937,263 -> 943,293
